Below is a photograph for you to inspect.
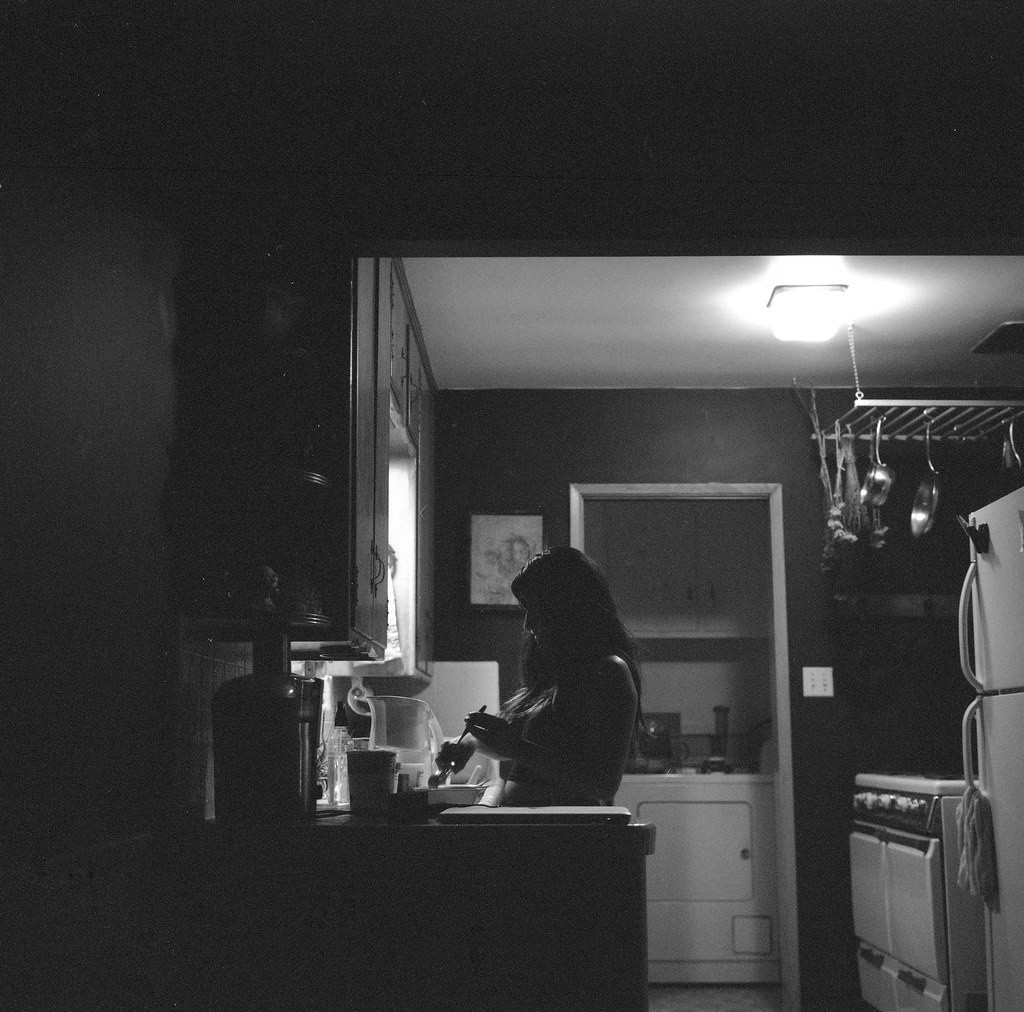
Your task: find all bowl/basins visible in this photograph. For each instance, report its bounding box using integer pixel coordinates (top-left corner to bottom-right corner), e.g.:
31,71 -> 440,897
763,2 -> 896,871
475,785 -> 490,804
413,786 -> 479,805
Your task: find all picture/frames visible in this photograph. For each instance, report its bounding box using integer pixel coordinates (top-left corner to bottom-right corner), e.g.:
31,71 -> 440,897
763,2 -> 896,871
461,507 -> 551,614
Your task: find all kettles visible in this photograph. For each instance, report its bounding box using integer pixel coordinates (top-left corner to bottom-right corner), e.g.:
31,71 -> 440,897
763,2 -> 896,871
366,695 -> 444,791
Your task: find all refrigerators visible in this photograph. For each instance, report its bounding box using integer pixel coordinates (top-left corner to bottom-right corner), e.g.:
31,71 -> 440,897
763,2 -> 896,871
959,484 -> 1024,1012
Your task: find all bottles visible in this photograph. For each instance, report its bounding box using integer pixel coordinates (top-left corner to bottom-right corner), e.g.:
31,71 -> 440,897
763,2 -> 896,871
327,726 -> 352,805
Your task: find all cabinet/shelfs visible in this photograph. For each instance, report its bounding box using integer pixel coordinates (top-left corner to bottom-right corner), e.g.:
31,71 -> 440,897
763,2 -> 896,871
584,501 -> 773,630
389,280 -> 428,452
180,259 -> 400,663
609,780 -> 777,985
401,364 -> 440,682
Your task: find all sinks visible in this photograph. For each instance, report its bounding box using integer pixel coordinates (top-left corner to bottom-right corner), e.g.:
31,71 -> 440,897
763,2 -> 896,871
414,786 -> 492,805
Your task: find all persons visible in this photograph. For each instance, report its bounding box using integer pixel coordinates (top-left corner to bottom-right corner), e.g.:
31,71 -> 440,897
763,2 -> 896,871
463,546 -> 647,810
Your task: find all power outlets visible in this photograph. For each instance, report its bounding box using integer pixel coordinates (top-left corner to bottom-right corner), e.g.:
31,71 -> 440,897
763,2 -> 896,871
802,667 -> 834,698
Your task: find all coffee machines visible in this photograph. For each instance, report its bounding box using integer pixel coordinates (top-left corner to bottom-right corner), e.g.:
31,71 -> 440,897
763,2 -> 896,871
212,674 -> 324,821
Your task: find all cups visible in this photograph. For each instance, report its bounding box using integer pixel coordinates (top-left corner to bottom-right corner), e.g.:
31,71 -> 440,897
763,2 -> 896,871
346,750 -> 396,817
635,711 -> 681,773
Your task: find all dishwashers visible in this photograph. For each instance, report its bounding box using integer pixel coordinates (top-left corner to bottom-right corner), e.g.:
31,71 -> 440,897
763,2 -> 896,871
849,772 -> 989,1012
